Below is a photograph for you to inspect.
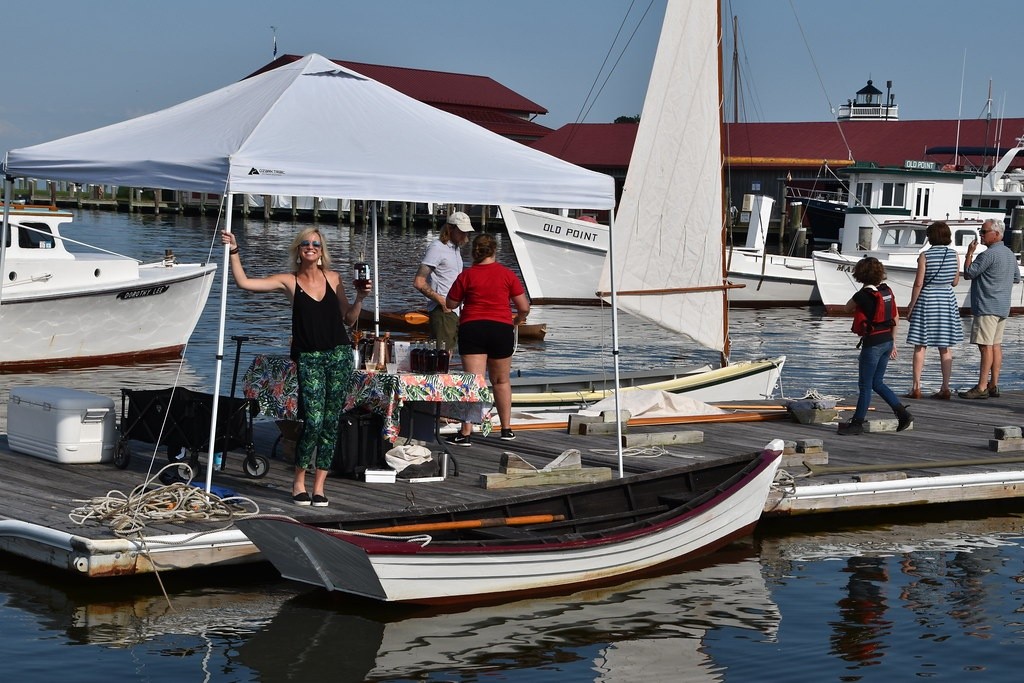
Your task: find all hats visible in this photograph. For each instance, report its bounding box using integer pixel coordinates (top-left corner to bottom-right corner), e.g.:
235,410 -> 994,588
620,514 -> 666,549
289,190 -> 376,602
447,211 -> 475,232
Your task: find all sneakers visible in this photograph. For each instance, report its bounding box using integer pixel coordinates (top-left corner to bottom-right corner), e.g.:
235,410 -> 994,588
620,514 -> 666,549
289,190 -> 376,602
500,428 -> 516,440
958,385 -> 990,398
987,382 -> 1000,397
443,431 -> 471,447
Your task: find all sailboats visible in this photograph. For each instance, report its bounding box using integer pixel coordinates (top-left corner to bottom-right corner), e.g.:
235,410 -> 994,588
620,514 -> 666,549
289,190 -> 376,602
432,0 -> 789,436
495,0 -> 859,310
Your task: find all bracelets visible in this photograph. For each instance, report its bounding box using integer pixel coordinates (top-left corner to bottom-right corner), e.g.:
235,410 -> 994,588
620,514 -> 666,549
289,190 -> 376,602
230,247 -> 238,255
965,254 -> 972,258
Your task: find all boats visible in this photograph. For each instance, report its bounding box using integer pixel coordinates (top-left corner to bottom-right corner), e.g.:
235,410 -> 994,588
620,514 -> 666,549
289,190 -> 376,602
810,47 -> 1024,318
228,438 -> 787,609
0,180 -> 218,375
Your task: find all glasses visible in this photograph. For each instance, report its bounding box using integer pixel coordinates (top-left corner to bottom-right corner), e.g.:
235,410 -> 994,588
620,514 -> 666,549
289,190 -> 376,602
301,240 -> 321,246
979,230 -> 995,234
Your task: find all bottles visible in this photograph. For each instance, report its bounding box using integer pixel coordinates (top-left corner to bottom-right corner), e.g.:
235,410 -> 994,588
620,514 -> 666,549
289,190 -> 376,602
359,332 -> 375,370
410,340 -> 450,374
354,252 -> 370,288
214,453 -> 222,467
383,332 -> 392,366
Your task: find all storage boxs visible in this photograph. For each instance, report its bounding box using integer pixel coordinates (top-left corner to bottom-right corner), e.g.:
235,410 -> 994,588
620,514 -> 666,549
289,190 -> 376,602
6,387 -> 117,463
363,468 -> 398,484
331,413 -> 391,476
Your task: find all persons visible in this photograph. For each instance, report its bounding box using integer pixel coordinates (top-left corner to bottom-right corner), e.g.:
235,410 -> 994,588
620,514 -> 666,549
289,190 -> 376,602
904,220 -> 965,401
837,258 -> 914,434
446,233 -> 530,447
220,225 -> 372,507
413,211 -> 475,361
958,218 -> 1021,399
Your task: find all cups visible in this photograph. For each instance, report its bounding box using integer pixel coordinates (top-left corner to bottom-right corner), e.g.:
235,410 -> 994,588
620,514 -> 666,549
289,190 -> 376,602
365,363 -> 377,371
386,363 -> 397,374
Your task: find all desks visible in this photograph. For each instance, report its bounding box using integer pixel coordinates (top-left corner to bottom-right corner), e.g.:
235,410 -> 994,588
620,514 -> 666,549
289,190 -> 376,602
242,352 -> 494,478
391,341 -> 446,372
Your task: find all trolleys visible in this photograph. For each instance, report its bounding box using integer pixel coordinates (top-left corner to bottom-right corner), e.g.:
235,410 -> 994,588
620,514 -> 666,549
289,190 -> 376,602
112,335 -> 270,483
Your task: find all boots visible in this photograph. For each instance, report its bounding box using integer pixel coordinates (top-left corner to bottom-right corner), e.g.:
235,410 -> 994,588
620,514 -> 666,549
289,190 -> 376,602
892,403 -> 914,432
837,416 -> 864,435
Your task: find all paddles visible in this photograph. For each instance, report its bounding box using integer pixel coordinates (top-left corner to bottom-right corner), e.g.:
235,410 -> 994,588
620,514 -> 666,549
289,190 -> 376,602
405,312 -> 526,325
333,515 -> 564,536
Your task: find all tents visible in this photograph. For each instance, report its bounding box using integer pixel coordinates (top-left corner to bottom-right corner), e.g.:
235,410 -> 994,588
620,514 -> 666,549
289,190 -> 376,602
0,53 -> 624,511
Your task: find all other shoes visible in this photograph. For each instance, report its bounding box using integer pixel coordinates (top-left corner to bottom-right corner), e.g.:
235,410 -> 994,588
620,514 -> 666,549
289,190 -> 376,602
312,492 -> 329,507
934,390 -> 951,400
292,484 -> 311,506
906,389 -> 920,399
